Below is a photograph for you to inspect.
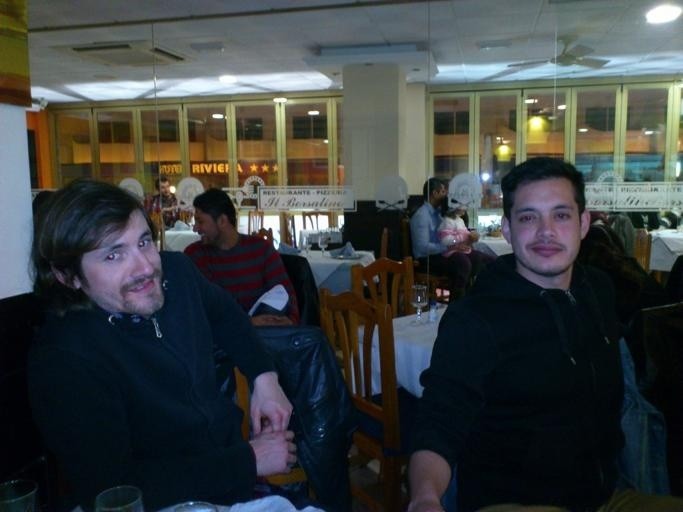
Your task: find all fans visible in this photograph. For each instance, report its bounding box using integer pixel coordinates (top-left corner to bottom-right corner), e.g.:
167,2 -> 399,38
507,31 -> 610,73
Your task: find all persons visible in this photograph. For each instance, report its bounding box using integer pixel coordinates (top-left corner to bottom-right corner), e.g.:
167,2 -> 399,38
410,176 -> 469,302
438,197 -> 494,283
182,187 -> 298,327
407,155 -> 683,512
23,178 -> 297,512
150,174 -> 182,227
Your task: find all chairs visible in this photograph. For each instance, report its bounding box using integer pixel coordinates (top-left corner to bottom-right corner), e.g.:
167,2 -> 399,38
248,211 -> 278,250
633,229 -> 652,277
302,211 -> 334,232
401,215 -> 452,318
361,227 -> 389,305
228,322 -> 329,505
318,287 -> 398,512
351,255 -> 417,327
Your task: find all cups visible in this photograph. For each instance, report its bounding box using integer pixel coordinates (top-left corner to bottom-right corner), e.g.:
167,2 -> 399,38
1,480 -> 39,512
174,502 -> 219,512
97,485 -> 144,511
303,237 -> 312,260
410,285 -> 428,326
318,236 -> 327,258
